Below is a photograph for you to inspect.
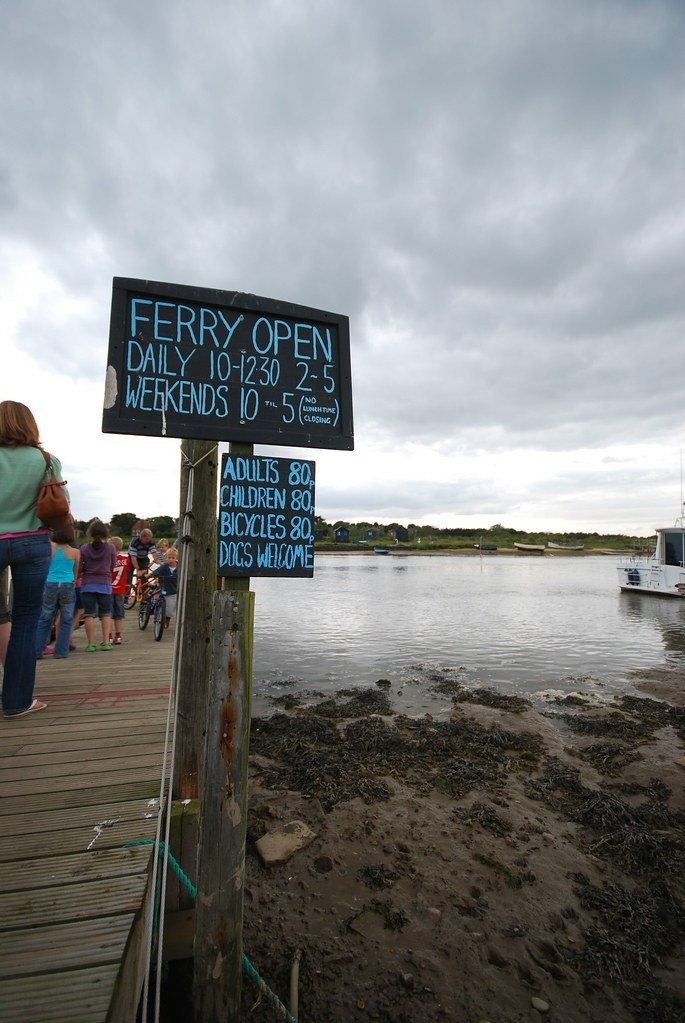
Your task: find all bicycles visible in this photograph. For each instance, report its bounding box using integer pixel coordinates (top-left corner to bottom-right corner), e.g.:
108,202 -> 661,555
123,565 -> 171,642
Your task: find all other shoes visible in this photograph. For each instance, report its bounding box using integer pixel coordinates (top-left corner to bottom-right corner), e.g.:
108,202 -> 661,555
123,599 -> 128,604
41,648 -> 54,655
109,638 -> 114,645
164,616 -> 170,629
85,644 -> 96,652
101,643 -> 112,651
114,637 -> 122,644
69,645 -> 75,651
36,654 -> 43,660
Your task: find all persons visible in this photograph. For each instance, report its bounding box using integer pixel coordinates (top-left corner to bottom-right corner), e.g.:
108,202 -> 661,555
0,399 -> 71,720
54,578 -> 82,650
36,525 -> 80,659
107,537 -> 130,645
79,522 -> 116,652
145,538 -> 179,628
125,529 -> 162,604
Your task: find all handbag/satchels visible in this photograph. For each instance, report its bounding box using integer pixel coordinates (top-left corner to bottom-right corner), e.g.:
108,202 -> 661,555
36,450 -> 69,529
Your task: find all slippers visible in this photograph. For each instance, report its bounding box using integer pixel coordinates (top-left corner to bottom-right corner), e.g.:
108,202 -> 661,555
3,698 -> 47,719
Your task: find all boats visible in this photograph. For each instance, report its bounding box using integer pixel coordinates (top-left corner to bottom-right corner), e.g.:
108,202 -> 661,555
373,546 -> 390,553
615,446 -> 685,598
513,542 -> 546,552
473,544 -> 497,550
547,541 -> 585,551
